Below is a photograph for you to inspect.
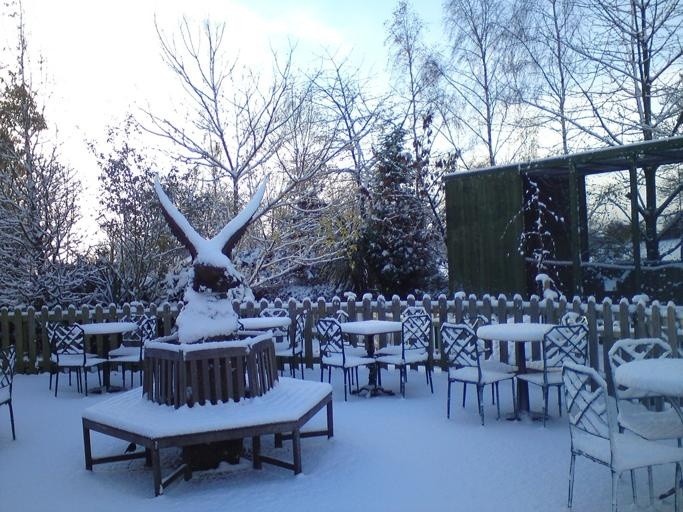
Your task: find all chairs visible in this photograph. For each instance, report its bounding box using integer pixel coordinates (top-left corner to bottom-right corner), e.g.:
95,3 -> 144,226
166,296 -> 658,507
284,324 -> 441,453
608,337 -> 682,477
441,322 -> 517,426
44,314 -> 158,397
317,318 -> 375,403
519,325 -> 589,427
525,312 -> 589,403
325,310 -> 368,385
377,308 -> 430,384
378,315 -> 433,396
274,312 -> 309,380
0,344 -> 16,440
561,363 -> 683,512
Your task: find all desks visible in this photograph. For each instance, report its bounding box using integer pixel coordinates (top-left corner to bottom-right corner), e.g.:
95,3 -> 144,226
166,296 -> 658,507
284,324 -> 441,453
615,358 -> 683,499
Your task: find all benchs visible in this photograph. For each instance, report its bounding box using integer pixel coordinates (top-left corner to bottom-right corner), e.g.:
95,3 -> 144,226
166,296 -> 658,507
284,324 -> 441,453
82,334 -> 334,497
